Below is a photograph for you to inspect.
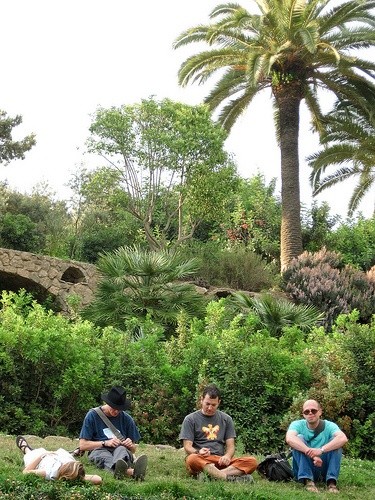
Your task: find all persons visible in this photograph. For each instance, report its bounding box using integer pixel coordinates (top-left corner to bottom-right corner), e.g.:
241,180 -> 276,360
15,435 -> 102,484
179,387 -> 258,483
79,384 -> 148,481
286,400 -> 347,493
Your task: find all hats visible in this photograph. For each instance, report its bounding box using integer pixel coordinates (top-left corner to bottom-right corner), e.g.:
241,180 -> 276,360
100,384 -> 131,408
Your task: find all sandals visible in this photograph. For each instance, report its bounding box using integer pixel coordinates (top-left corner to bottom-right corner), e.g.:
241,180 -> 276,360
70,447 -> 85,458
16,437 -> 32,454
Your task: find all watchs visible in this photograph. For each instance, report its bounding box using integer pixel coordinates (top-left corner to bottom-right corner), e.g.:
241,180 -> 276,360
320,447 -> 325,453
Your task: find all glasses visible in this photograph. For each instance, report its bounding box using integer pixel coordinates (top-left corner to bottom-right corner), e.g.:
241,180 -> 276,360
106,403 -> 127,412
302,409 -> 319,416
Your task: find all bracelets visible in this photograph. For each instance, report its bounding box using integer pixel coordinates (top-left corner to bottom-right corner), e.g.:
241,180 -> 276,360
102,440 -> 105,447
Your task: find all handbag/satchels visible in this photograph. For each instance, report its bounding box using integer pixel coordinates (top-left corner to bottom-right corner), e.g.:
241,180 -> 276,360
256,453 -> 293,482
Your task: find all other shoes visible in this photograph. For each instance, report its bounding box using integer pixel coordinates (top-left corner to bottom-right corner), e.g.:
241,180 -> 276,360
195,472 -> 205,483
113,459 -> 128,480
232,474 -> 252,484
328,482 -> 337,494
133,454 -> 147,481
305,483 -> 318,493
